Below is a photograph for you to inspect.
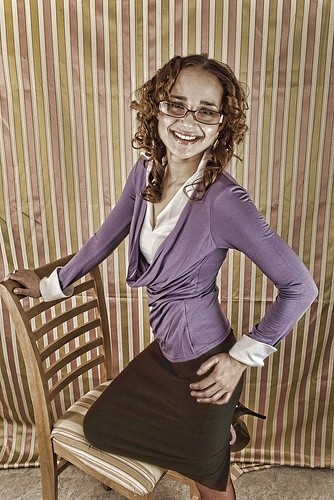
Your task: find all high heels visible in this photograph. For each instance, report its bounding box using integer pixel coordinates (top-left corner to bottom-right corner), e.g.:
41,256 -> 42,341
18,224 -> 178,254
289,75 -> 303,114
228,401 -> 266,453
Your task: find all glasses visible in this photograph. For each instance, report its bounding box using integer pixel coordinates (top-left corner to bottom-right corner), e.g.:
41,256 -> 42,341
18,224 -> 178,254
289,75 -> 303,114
158,101 -> 224,126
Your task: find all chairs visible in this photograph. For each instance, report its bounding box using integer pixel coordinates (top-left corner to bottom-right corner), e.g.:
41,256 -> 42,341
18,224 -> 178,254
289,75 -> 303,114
0,252 -> 169,499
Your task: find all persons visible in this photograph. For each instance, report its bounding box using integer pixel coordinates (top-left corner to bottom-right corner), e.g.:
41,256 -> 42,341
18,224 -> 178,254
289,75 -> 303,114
3,54 -> 319,500
